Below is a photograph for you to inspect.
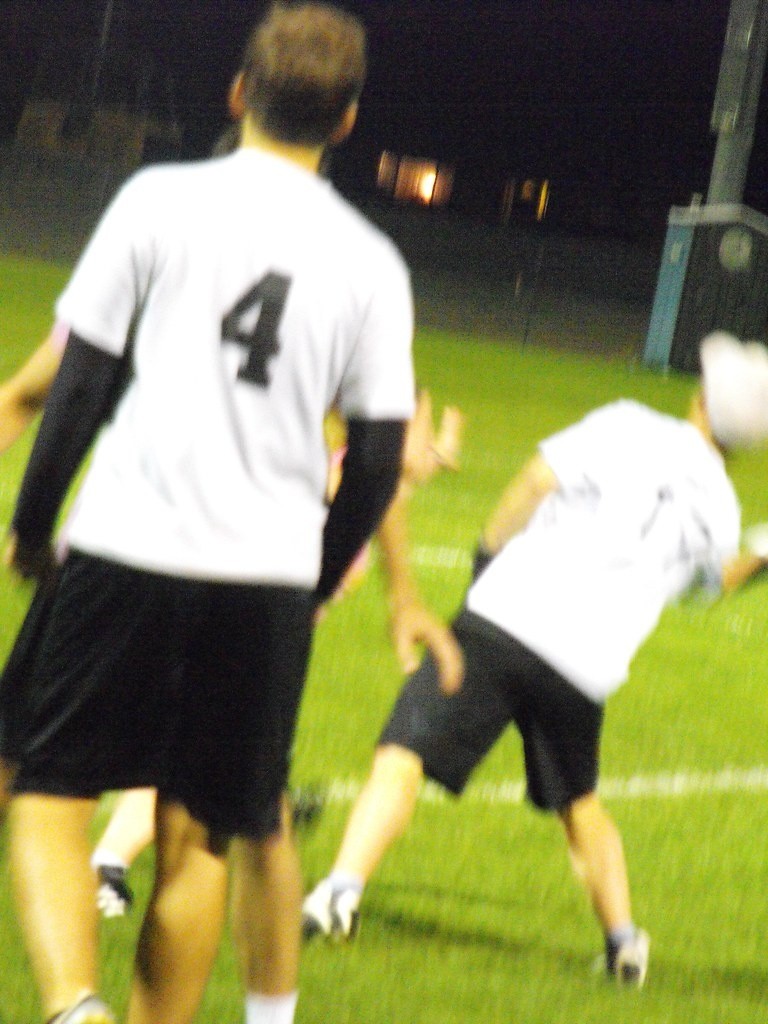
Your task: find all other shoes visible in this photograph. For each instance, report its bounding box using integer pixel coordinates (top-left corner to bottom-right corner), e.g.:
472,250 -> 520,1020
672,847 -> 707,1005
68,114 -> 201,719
301,877 -> 358,946
93,855 -> 132,920
48,995 -> 113,1024
605,930 -> 646,991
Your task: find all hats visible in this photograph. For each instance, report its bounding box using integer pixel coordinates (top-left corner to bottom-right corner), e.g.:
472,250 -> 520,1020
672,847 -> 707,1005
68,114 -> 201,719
700,332 -> 768,445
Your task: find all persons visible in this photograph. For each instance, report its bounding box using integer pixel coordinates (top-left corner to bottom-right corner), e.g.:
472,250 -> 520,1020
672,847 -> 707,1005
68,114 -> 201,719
0,9 -> 466,1024
298,331 -> 768,986
